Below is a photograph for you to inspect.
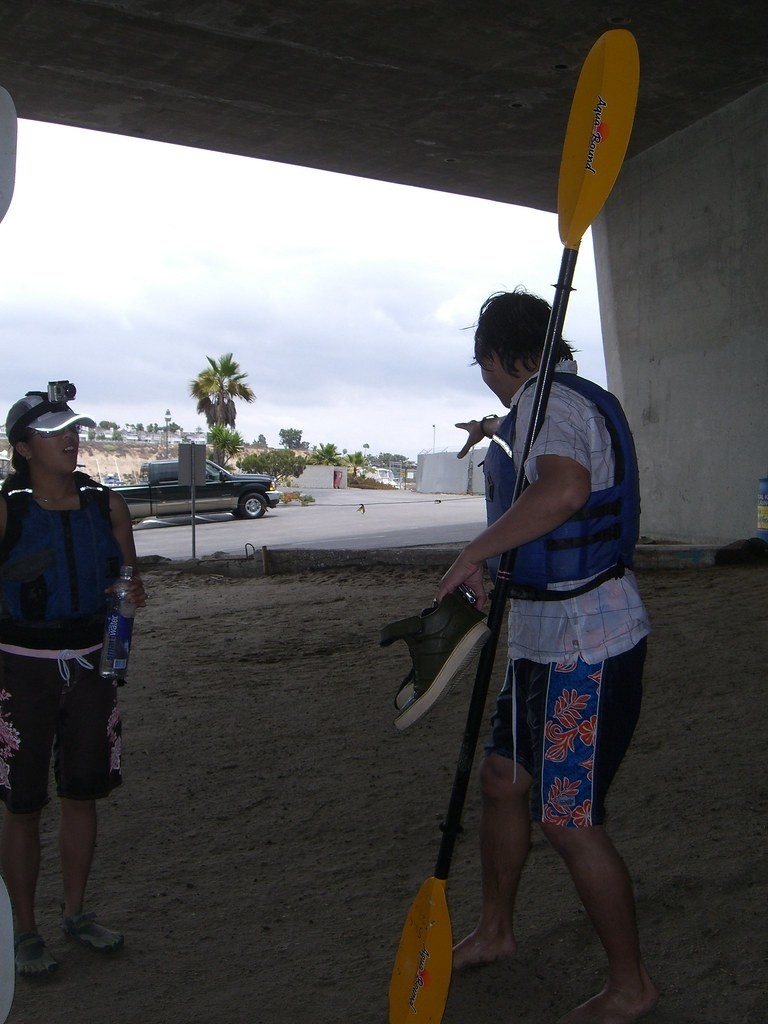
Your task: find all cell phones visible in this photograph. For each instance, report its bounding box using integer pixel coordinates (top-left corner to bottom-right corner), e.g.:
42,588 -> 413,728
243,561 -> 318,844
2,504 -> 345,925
458,583 -> 478,604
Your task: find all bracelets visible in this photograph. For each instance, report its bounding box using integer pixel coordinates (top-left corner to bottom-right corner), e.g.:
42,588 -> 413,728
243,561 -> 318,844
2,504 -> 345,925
478,413 -> 498,440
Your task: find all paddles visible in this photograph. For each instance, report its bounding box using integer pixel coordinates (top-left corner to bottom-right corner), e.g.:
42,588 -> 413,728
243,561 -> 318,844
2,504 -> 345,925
388,28 -> 640,1024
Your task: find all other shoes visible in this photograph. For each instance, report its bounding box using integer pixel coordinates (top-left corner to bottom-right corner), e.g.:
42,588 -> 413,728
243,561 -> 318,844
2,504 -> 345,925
15,933 -> 59,977
59,903 -> 124,950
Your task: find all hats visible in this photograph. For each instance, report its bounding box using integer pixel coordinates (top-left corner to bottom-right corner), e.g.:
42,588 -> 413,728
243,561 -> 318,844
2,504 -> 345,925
7,391 -> 96,436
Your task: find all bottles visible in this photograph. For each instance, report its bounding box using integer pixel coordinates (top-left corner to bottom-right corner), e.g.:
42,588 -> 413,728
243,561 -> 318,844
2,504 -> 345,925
98,566 -> 135,678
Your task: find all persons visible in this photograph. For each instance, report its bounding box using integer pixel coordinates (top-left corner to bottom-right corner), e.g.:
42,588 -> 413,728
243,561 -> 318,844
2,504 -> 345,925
0,390 -> 148,978
430,288 -> 663,1024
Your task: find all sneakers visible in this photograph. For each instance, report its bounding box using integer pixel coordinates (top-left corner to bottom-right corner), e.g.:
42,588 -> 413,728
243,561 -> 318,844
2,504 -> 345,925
379,592 -> 491,731
394,588 -> 489,709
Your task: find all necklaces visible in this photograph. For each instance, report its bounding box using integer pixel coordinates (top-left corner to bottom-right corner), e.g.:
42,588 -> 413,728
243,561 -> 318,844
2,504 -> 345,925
32,489 -> 78,503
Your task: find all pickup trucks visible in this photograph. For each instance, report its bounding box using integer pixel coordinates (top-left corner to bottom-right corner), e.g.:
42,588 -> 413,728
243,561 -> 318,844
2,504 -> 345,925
111,458 -> 280,527
